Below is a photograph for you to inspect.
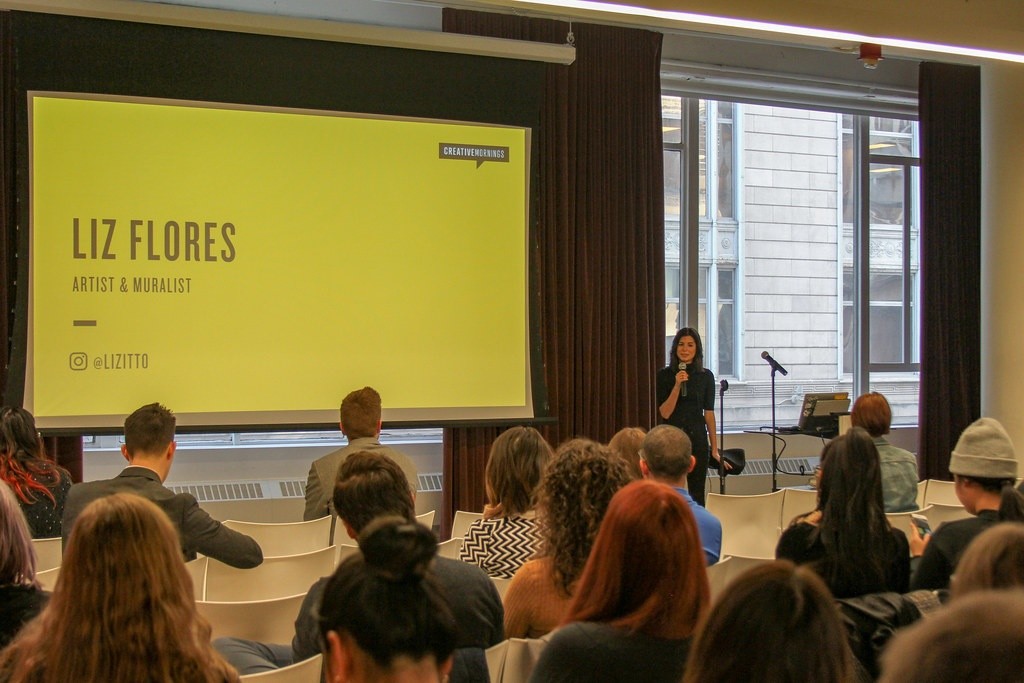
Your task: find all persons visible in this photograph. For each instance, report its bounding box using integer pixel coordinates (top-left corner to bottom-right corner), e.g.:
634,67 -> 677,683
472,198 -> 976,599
317,515 -> 459,683
531,479 -> 710,683
0,479 -> 56,649
210,451 -> 506,676
500,440 -> 630,640
908,418 -> 1024,590
953,521 -> 1024,599
880,586 -> 1024,683
656,327 -> 731,509
63,402 -> 263,569
457,425 -> 556,579
776,428 -> 910,599
850,391 -> 920,513
609,427 -> 647,479
640,425 -> 722,567
691,559 -> 857,683
303,384 -> 417,521
0,406 -> 72,539
0,495 -> 242,683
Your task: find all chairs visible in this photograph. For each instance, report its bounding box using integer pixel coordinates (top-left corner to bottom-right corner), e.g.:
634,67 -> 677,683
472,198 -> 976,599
437,509 -> 484,559
486,577 -> 547,683
225,515 -> 333,556
339,544 -> 360,559
234,653 -> 322,683
183,557 -> 206,601
31,533 -> 62,592
192,593 -> 307,645
206,546 -> 336,599
333,510 -> 435,544
705,478 -> 973,600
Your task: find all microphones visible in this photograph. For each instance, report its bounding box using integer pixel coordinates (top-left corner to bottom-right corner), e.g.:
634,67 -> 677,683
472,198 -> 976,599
679,362 -> 687,396
761,351 -> 788,376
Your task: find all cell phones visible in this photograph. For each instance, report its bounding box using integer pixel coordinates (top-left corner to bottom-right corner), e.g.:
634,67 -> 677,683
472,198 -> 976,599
910,514 -> 932,539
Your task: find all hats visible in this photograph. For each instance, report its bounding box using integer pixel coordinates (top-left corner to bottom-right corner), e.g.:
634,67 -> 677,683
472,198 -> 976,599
949,417 -> 1019,479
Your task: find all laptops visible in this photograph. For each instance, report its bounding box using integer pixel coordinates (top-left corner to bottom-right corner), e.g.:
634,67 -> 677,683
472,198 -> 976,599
778,391 -> 851,432
